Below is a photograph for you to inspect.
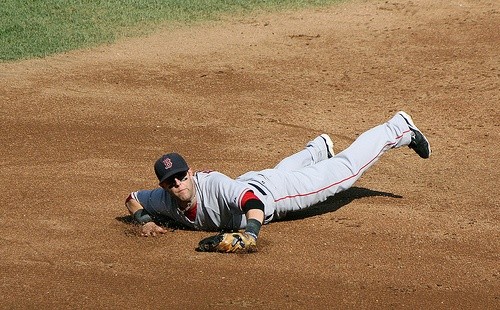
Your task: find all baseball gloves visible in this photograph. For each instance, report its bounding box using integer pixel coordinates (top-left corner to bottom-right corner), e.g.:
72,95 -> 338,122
198,232 -> 258,253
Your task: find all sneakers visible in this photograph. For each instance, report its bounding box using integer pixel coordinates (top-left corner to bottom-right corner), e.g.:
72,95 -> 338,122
320,134 -> 334,158
396,111 -> 431,159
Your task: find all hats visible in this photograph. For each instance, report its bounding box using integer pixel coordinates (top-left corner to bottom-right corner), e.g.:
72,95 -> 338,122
154,153 -> 189,186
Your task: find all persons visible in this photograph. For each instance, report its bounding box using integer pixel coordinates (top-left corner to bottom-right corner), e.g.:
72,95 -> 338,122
125,111 -> 432,253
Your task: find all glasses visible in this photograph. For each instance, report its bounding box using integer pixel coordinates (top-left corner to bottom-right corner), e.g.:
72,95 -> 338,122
164,171 -> 190,189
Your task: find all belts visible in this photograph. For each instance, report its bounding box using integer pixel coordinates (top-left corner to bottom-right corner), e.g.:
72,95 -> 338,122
249,182 -> 273,220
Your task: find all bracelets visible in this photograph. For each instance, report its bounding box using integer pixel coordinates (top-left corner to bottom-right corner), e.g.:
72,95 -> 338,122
133,208 -> 153,226
245,218 -> 262,239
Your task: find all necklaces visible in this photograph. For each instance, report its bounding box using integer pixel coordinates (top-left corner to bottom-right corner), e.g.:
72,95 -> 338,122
176,196 -> 197,215
